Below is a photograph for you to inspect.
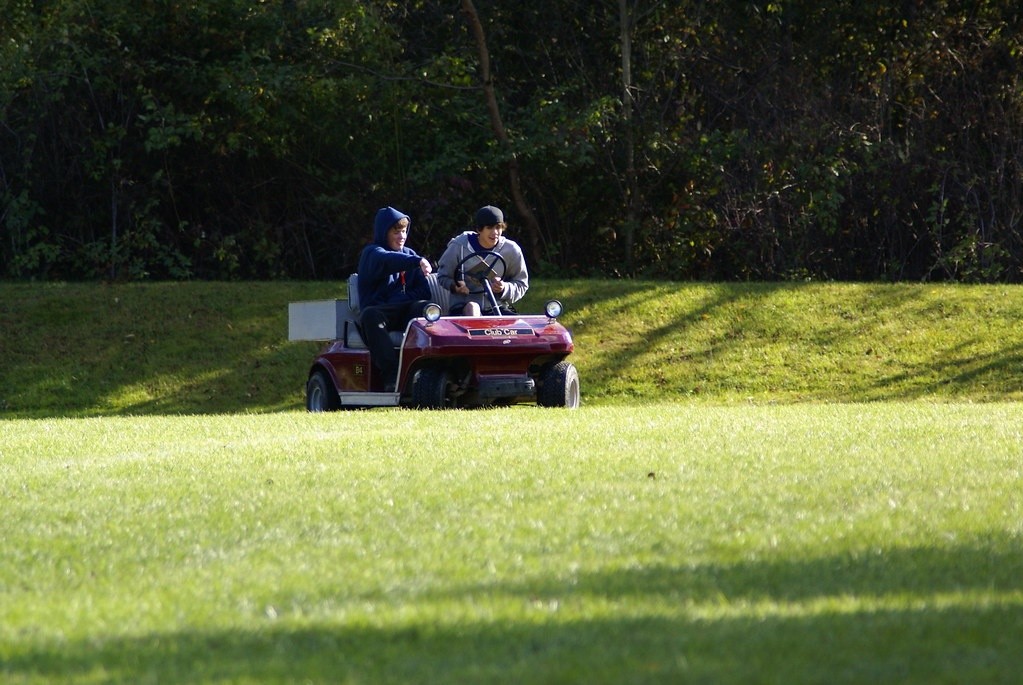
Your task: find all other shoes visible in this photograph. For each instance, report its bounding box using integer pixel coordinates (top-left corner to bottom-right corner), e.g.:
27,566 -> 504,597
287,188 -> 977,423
383,374 -> 395,391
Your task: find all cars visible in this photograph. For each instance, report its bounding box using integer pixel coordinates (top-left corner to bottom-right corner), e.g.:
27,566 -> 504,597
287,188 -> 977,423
306,249 -> 581,412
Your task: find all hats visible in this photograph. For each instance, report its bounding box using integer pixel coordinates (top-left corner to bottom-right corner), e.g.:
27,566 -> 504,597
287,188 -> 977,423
477,205 -> 504,225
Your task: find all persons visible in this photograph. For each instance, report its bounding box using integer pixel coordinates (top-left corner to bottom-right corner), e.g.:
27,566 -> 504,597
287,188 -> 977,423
437,205 -> 529,316
357,206 -> 433,382
